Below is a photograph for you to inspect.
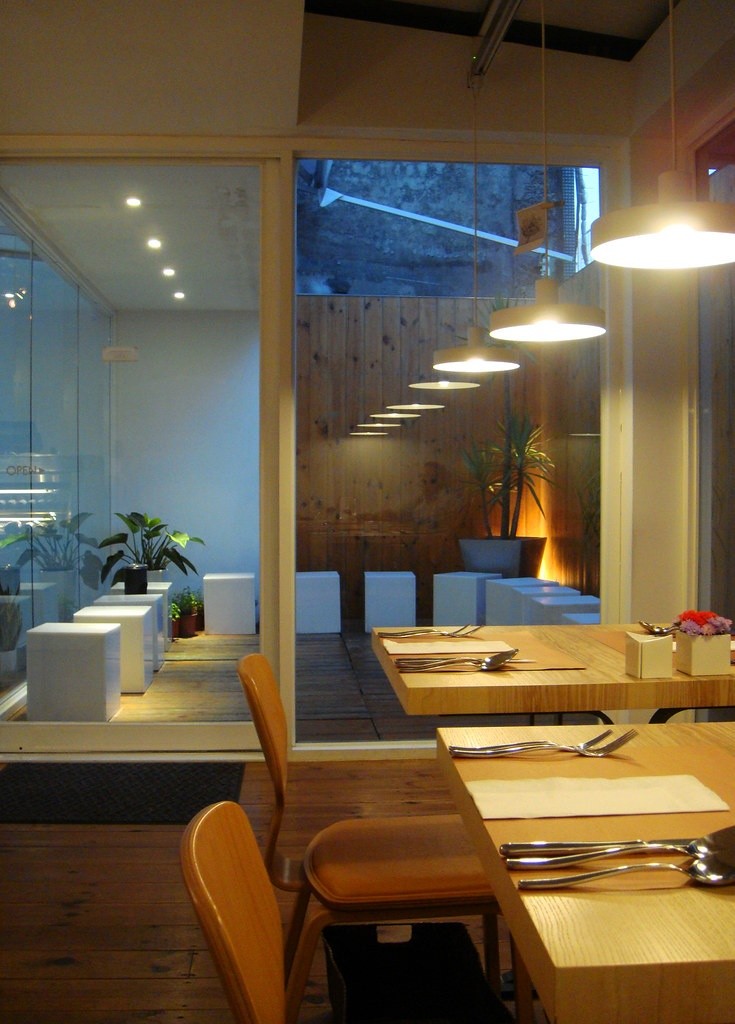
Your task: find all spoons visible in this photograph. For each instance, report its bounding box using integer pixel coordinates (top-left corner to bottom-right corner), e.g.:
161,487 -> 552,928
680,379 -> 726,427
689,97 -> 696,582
398,648 -> 519,672
638,621 -> 680,634
517,850 -> 735,888
506,826 -> 735,870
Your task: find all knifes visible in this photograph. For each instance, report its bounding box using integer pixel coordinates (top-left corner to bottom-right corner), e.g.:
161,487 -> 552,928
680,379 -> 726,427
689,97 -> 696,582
499,838 -> 699,858
397,657 -> 537,662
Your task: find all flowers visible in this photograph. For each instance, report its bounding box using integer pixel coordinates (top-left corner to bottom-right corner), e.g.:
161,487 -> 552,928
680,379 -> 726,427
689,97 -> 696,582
676,609 -> 733,637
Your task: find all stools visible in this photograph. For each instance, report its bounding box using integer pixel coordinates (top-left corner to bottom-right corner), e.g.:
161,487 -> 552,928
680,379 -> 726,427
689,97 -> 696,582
562,613 -> 600,625
531,596 -> 600,625
20,582 -> 59,625
72,605 -> 154,694
512,585 -> 581,625
0,595 -> 39,646
110,581 -> 172,652
432,571 -> 503,627
296,570 -> 342,634
203,573 -> 257,635
93,593 -> 165,672
363,571 -> 417,632
485,578 -> 559,632
26,622 -> 122,721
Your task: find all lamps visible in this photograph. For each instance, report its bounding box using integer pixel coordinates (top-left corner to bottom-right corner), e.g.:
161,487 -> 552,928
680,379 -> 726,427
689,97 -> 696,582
431,79 -> 523,374
590,0 -> 735,271
487,0 -> 608,344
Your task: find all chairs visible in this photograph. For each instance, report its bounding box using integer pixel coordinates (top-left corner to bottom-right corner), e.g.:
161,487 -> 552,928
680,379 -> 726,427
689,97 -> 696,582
237,653 -> 538,1024
178,800 -> 288,1024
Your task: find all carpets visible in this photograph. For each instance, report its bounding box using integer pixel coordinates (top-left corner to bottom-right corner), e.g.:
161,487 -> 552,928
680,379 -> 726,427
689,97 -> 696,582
0,762 -> 247,826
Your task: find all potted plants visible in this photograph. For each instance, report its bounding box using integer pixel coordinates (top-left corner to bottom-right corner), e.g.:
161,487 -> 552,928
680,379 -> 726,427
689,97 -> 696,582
98,511 -> 206,587
168,585 -> 203,639
0,599 -> 21,676
451,290 -> 559,577
0,511 -> 104,622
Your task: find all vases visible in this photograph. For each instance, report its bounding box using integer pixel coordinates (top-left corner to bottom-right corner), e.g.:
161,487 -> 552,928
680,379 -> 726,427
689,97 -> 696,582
626,633 -> 673,679
675,629 -> 731,677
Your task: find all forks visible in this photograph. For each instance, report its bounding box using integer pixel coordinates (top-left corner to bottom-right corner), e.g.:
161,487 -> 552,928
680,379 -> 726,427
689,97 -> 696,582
451,729 -> 638,757
378,624 -> 486,638
449,729 -> 613,752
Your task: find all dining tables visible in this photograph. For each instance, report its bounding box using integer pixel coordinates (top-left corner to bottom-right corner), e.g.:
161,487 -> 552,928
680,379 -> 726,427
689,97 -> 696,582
434,721 -> 735,1024
370,623 -> 735,725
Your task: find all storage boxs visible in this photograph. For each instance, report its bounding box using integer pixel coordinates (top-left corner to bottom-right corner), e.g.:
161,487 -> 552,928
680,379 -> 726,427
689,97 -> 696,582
323,922 -> 515,1024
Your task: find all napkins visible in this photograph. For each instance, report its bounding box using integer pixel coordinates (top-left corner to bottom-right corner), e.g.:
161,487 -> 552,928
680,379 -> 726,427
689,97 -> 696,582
463,774 -> 731,822
382,638 -> 515,655
